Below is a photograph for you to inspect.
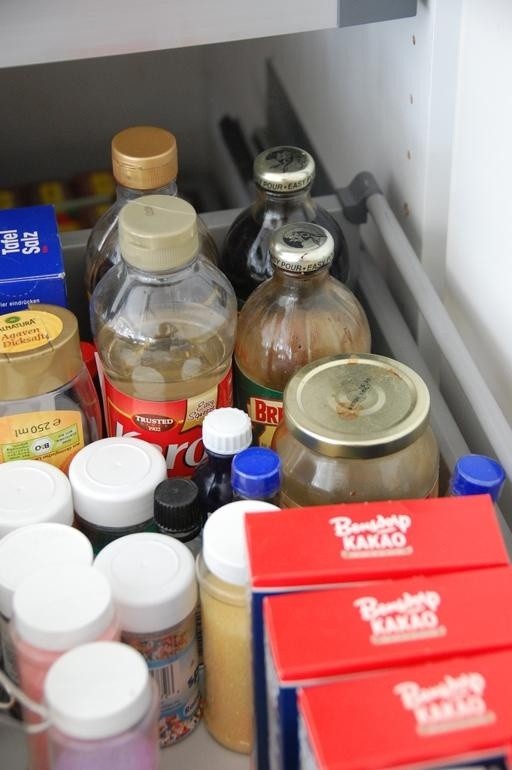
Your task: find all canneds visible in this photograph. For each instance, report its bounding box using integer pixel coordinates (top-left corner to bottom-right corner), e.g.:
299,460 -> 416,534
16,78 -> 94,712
0,304 -> 102,468
271,351 -> 438,514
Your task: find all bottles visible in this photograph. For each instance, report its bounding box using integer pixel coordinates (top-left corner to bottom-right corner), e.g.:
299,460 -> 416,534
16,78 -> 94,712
1,124 -> 512,770
